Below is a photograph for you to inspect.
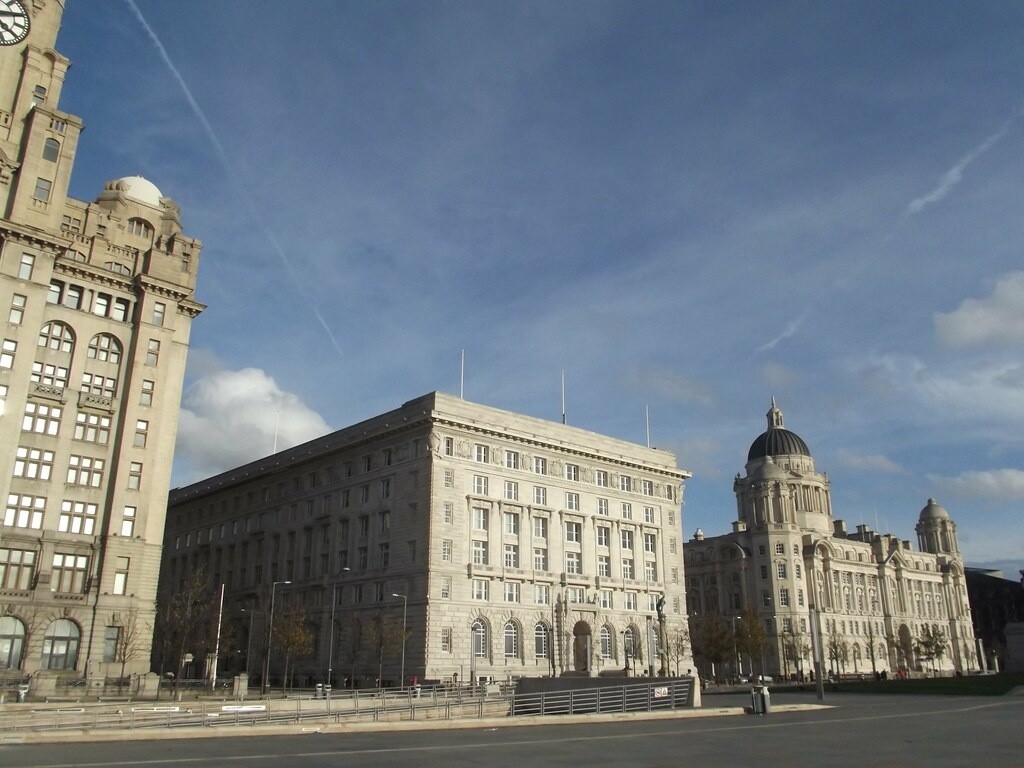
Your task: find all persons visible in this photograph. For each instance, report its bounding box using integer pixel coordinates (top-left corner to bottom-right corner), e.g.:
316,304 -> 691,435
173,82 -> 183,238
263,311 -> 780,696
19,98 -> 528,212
641,670 -> 649,676
876,668 -> 887,680
829,669 -> 833,675
900,670 -> 905,679
685,669 -> 692,677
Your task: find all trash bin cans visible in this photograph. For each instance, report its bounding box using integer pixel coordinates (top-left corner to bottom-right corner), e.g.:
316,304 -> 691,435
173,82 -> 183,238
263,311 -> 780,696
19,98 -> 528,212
17,683 -> 29,702
414,684 -> 421,698
751,685 -> 770,714
315,683 -> 332,699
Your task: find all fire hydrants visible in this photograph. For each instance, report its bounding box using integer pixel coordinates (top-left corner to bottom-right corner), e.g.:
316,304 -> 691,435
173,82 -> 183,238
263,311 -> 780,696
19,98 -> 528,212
411,676 -> 418,689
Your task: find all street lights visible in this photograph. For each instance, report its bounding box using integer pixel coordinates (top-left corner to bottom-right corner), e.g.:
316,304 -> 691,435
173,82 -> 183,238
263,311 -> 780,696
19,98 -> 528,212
732,616 -> 741,674
393,594 -> 407,692
327,567 -> 350,685
551,581 -> 567,677
264,580 -> 292,692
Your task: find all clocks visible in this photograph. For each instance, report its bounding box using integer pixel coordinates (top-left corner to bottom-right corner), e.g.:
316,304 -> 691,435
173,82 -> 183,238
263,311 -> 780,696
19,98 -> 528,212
0,0 -> 31,47
